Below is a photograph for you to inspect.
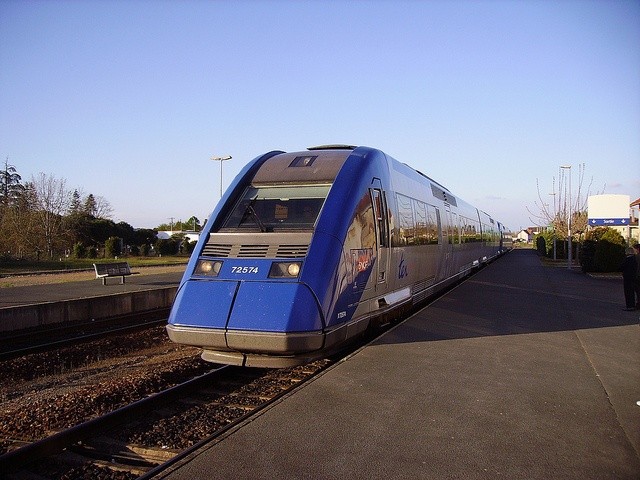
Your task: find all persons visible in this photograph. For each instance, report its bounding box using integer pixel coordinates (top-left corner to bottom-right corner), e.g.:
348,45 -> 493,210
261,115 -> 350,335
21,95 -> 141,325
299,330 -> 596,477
296,204 -> 315,224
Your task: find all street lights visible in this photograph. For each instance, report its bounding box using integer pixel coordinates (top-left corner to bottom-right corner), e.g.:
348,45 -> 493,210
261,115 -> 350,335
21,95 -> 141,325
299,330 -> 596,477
212,156 -> 232,198
548,194 -> 556,261
561,166 -> 571,271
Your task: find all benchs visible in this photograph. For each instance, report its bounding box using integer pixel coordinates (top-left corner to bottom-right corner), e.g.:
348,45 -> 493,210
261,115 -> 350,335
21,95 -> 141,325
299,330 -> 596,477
93,262 -> 140,285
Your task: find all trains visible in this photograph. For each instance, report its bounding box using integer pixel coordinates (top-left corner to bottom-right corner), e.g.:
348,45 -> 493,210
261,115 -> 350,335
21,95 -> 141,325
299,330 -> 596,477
166,144 -> 512,368
